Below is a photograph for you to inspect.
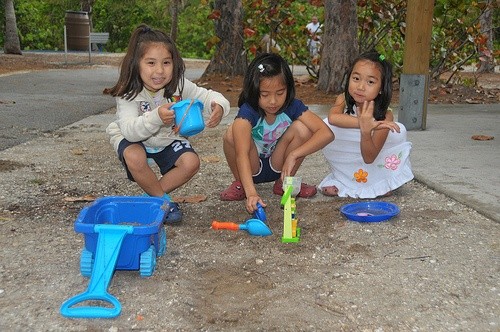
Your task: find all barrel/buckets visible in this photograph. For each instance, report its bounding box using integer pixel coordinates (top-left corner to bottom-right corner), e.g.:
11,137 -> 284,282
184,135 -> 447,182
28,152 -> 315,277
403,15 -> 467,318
169,99 -> 204,136
63,9 -> 90,52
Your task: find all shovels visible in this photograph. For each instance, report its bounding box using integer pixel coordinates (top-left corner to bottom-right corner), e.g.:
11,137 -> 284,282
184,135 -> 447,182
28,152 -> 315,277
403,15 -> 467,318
212,219 -> 273,237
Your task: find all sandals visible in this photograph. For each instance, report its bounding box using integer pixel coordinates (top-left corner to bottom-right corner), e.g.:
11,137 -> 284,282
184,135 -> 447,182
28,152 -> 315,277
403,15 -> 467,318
273,181 -> 316,197
220,181 -> 247,202
164,201 -> 183,223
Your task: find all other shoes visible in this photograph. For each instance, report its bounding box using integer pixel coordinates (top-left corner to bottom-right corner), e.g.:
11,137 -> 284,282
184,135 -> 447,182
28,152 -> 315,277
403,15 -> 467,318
321,186 -> 339,196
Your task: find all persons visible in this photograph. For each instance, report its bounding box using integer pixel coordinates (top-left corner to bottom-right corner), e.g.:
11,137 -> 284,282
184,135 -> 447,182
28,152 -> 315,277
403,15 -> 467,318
219,52 -> 336,215
102,23 -> 230,225
317,50 -> 414,200
